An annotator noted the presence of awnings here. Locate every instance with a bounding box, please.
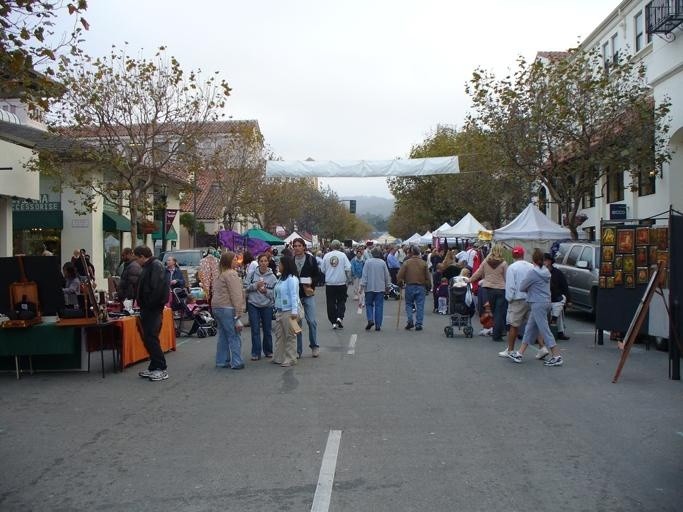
[137,220,178,241]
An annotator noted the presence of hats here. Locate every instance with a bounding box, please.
[512,246,526,257]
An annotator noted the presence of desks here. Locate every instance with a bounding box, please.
[0,302,176,382]
[591,285,668,353]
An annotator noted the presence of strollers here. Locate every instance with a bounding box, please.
[444,275,474,338]
[433,276,450,315]
[383,281,399,300]
[172,287,216,338]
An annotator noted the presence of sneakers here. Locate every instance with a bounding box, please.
[499,348,513,357]
[508,351,522,363]
[365,320,382,331]
[216,346,320,370]
[138,369,169,381]
[405,322,423,330]
[536,346,549,359]
[543,356,564,366]
[332,317,343,329]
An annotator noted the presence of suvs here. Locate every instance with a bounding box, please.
[161,246,217,289]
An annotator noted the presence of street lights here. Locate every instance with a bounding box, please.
[159,182,167,252]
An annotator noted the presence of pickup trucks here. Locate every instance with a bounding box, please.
[550,241,643,322]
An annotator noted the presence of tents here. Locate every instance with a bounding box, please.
[376,202,571,245]
[284,231,313,248]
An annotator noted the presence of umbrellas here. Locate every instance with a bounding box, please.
[238,226,285,247]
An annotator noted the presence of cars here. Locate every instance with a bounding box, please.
[271,243,293,265]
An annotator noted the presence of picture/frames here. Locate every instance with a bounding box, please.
[595,223,671,292]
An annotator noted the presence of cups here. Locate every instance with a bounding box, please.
[259,276,264,289]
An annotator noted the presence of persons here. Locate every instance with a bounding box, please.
[130,245,172,383]
[113,248,140,311]
[199,238,320,370]
[32,242,53,256]
[499,247,570,367]
[166,257,214,323]
[315,238,511,343]
[61,249,97,308]
